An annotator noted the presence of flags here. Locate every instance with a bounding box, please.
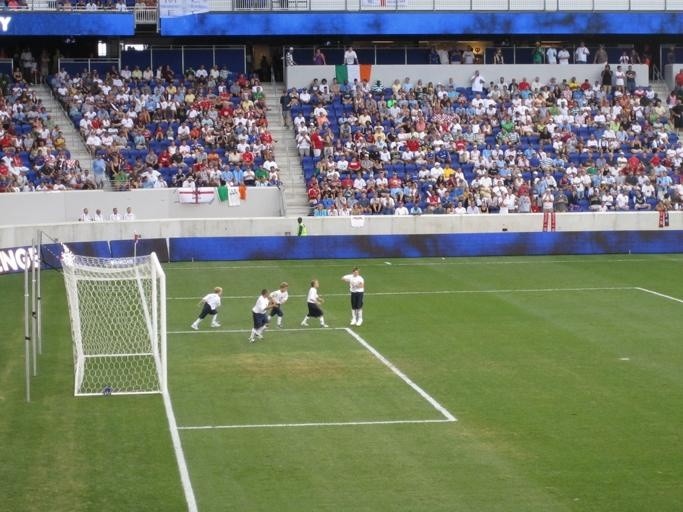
[217,185,247,201]
[177,186,216,204]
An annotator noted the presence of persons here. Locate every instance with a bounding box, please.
[267,282,288,329]
[123,207,136,220]
[189,286,222,329]
[280,65,682,216]
[1,1,159,21]
[78,207,93,222]
[248,288,278,343]
[296,217,308,236]
[300,278,330,330]
[1,46,284,193]
[109,207,122,221]
[341,268,365,326]
[92,209,105,221]
[286,40,678,65]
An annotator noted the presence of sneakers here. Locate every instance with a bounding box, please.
[350,319,363,326]
[248,333,265,343]
[321,324,328,328]
[211,322,220,327]
[277,324,282,327]
[301,321,309,327]
[191,323,199,329]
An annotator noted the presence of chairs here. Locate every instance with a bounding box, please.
[1,68,274,190]
[286,83,683,217]
